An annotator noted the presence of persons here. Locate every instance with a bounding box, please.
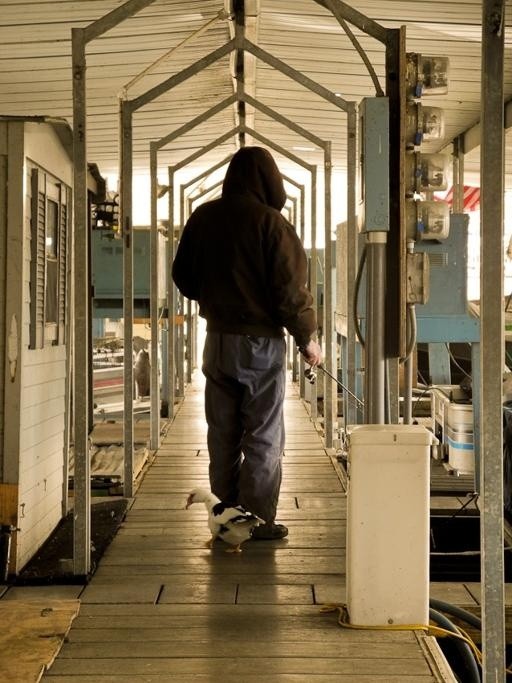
[171,146,325,540]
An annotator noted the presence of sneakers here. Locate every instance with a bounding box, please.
[253,523,288,540]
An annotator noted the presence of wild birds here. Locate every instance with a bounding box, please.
[184,486,264,553]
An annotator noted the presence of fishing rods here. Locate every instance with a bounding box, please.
[298,347,365,410]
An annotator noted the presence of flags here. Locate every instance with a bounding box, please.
[444,181,481,210]
